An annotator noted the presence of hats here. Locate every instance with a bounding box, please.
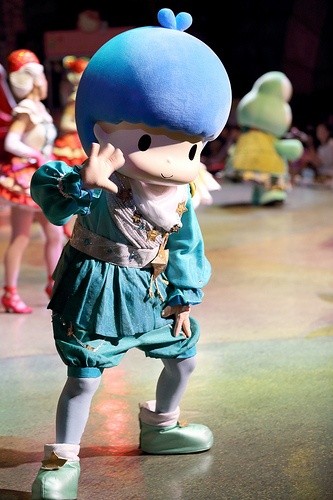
[8,49,39,71]
[64,55,90,74]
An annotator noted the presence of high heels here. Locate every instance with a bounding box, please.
[2,286,31,313]
[45,275,54,299]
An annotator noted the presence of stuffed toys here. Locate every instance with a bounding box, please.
[30,8,214,500]
[225,71,304,203]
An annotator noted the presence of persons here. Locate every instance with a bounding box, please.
[2,50,63,314]
[53,56,85,236]
[306,124,333,180]
[204,127,313,182]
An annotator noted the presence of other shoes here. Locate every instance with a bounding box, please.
[32,444,81,500]
[253,189,284,204]
[139,401,214,453]
